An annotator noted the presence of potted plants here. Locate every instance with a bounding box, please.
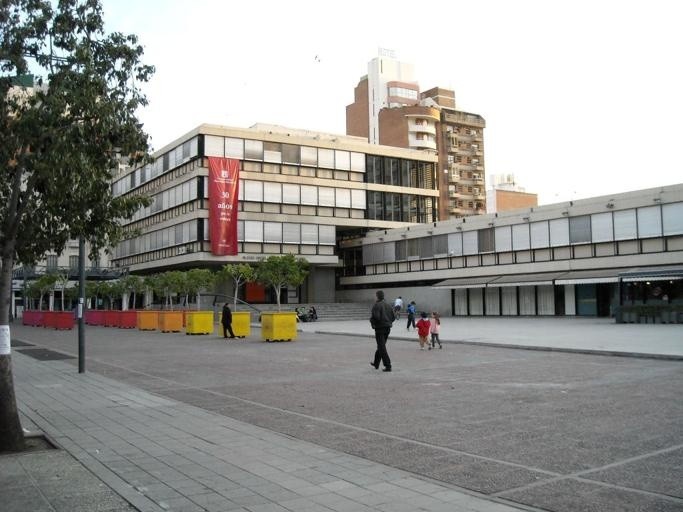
[19,251,310,342]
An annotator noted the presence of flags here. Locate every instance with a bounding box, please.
[207,155,242,259]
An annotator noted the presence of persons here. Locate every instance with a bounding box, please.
[406,301,417,331]
[415,311,432,350]
[370,290,396,373]
[296,306,308,322]
[429,312,443,349]
[221,302,237,338]
[394,296,403,320]
[308,306,317,322]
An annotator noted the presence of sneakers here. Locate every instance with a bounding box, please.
[419,344,442,350]
[383,368,390,372]
[371,363,378,369]
